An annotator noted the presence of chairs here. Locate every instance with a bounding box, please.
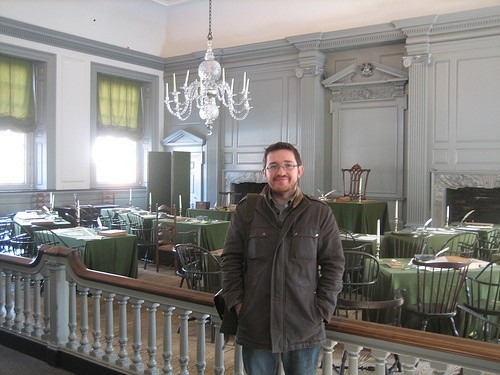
[0,164,500,375]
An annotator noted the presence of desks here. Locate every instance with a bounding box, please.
[56,205,119,227]
[185,208,233,221]
[320,198,390,235]
[442,222,500,250]
[33,228,138,279]
[99,208,178,243]
[149,217,231,266]
[363,254,500,343]
[12,212,72,235]
[339,233,388,266]
[387,221,479,258]
[202,249,224,294]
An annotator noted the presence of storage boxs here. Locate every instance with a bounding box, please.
[196,202,210,210]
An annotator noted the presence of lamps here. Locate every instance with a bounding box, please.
[164,0,251,134]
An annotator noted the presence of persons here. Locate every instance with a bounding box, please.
[222,142,345,375]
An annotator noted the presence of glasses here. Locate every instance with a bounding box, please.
[265,162,299,172]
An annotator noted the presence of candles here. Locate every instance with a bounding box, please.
[50,192,52,203]
[179,194,182,208]
[129,188,132,200]
[377,219,380,244]
[77,200,80,217]
[149,192,152,204]
[52,195,55,208]
[394,200,398,218]
[359,178,362,194]
[446,206,449,217]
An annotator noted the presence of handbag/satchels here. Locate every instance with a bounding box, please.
[214,288,225,320]
